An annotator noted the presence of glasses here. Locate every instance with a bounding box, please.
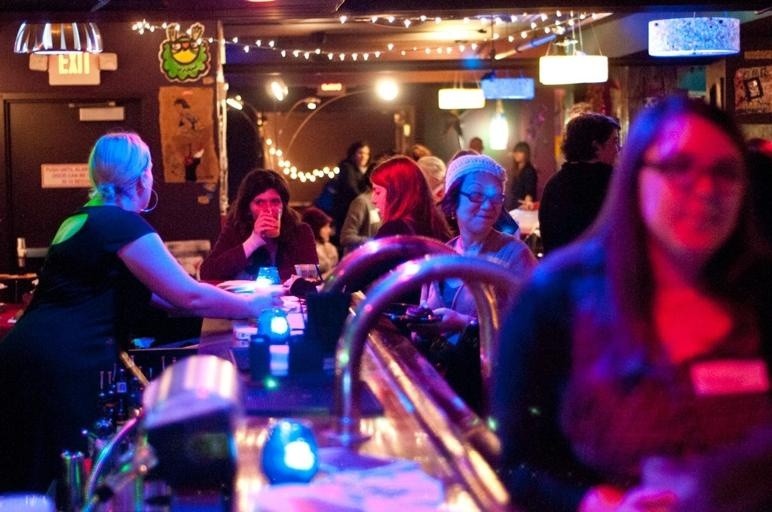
[641,158,746,192]
[459,190,507,205]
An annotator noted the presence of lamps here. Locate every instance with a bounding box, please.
[647,12,740,57]
[437,34,485,110]
[375,77,398,101]
[13,20,103,55]
[481,17,535,100]
[265,76,287,101]
[538,13,608,86]
[316,80,346,97]
[304,98,320,110]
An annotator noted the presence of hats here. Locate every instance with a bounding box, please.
[301,207,333,243]
[439,151,508,199]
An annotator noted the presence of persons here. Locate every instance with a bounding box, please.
[173,97,207,183]
[400,150,542,420]
[537,112,622,257]
[199,168,319,286]
[509,142,537,211]
[340,154,396,258]
[286,157,455,338]
[470,137,485,154]
[408,145,430,164]
[416,155,460,237]
[330,141,373,262]
[1,129,288,492]
[300,207,340,281]
[487,95,770,511]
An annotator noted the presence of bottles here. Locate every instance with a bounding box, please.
[257,307,290,344]
[258,266,282,284]
[259,413,321,485]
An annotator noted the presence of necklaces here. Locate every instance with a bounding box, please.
[459,236,482,253]
